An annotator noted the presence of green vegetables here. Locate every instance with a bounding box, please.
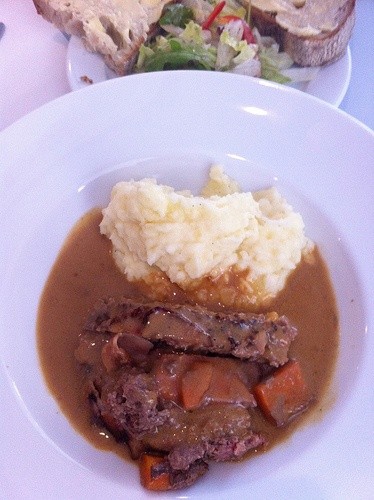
[133,0,291,84]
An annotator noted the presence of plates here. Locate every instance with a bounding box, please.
[0,70,374,498]
[65,33,353,109]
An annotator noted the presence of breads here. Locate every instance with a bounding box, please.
[31,0,175,75]
[238,0,355,66]
